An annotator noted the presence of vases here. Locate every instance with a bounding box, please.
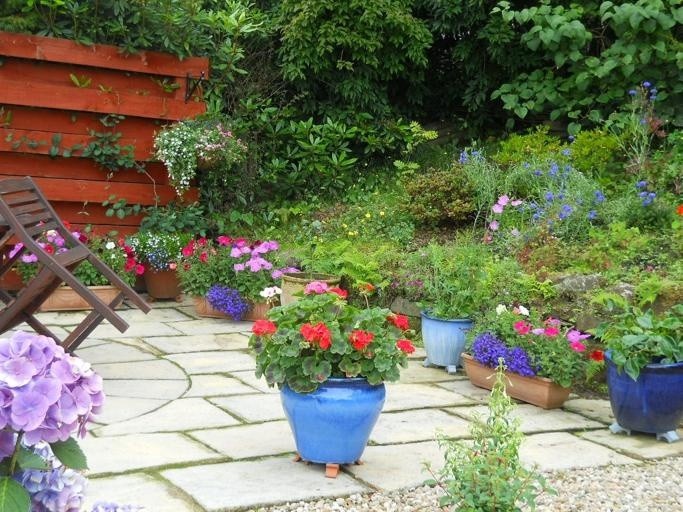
[460,352,571,408]
[197,156,222,169]
[279,377,385,464]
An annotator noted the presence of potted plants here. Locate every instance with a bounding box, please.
[585,293,683,443]
[408,230,498,374]
[281,223,390,314]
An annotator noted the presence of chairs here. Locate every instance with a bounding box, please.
[0,176,151,358]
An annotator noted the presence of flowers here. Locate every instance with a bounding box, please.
[472,302,605,385]
[154,113,250,198]
[248,281,415,394]
[8,221,300,320]
